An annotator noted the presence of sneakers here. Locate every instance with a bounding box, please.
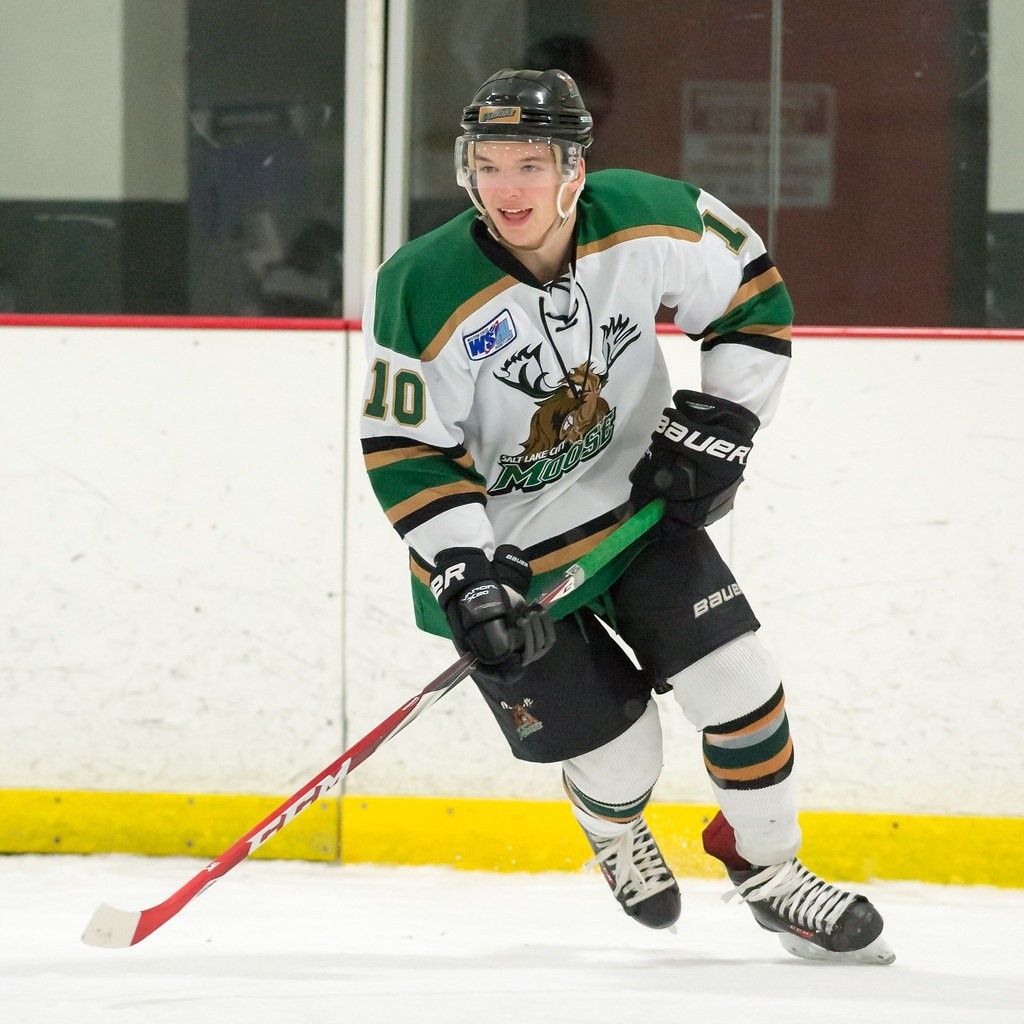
[703,807,895,967]
[567,790,682,935]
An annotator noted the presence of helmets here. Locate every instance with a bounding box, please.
[460,67,594,152]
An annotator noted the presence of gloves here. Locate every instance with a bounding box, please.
[630,388,761,544]
[428,542,553,687]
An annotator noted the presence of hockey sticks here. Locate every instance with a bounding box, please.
[75,491,670,962]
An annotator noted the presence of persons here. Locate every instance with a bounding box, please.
[359,68,885,956]
